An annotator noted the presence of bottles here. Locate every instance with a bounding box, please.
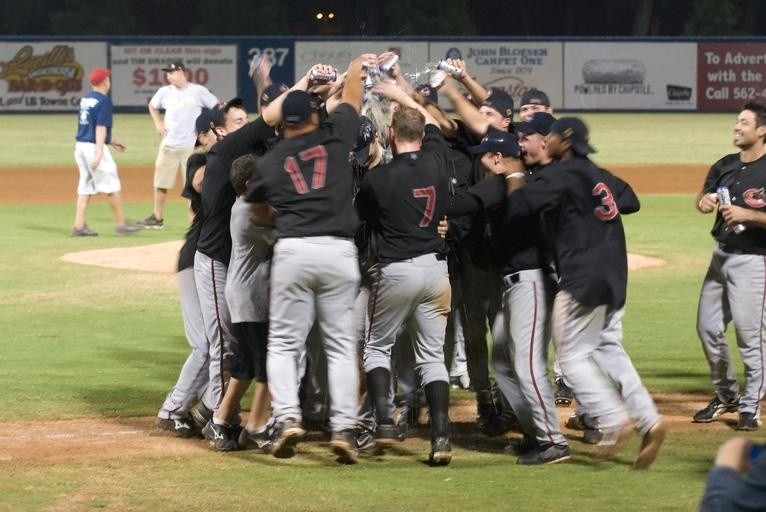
[373,64,380,77]
[311,68,336,81]
[381,55,399,71]
[364,75,373,86]
[732,222,745,234]
[437,59,464,79]
[717,186,732,207]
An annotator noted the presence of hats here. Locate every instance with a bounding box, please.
[281,90,325,126]
[520,90,550,107]
[351,115,375,164]
[89,68,112,86]
[260,83,289,107]
[416,83,439,104]
[208,97,243,135]
[196,112,220,134]
[479,87,514,119]
[162,62,185,71]
[509,112,556,135]
[469,131,521,157]
[551,117,596,156]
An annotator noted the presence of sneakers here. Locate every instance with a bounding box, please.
[737,412,762,430]
[633,420,667,469]
[556,385,572,408]
[155,409,196,438]
[396,402,418,427]
[239,427,276,453]
[331,429,355,466]
[194,407,214,427]
[271,418,306,459]
[72,224,97,237]
[201,420,237,451]
[357,433,375,451]
[137,214,165,232]
[478,405,495,430]
[692,398,742,424]
[485,410,517,435]
[505,436,540,455]
[429,435,453,465]
[113,223,144,233]
[517,443,571,466]
[593,423,636,462]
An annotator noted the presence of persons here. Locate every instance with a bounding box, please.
[358,82,457,467]
[252,53,378,466]
[136,60,217,230]
[513,110,605,444]
[199,153,296,455]
[428,58,523,434]
[445,129,573,468]
[193,63,338,442]
[151,108,221,440]
[505,116,669,472]
[692,101,766,432]
[518,89,556,130]
[343,107,395,439]
[71,67,144,236]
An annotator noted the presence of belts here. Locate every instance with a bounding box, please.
[409,252,448,263]
[720,243,764,258]
[501,274,524,288]
